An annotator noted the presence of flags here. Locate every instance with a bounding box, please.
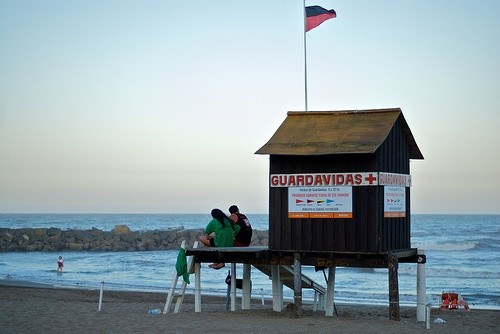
[306,5,336,32]
[175,248,191,284]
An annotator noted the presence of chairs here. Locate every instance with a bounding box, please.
[438,290,465,310]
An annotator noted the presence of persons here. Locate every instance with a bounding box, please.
[200,209,240,247]
[209,206,252,270]
[57,256,64,271]
